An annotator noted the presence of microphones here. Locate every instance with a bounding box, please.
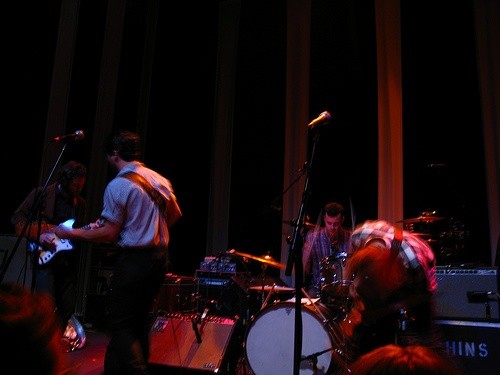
[51,131,85,143]
[192,324,203,343]
[308,111,332,129]
[466,290,496,301]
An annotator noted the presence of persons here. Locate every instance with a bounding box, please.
[336,219,438,335]
[301,204,357,298]
[13,162,93,327]
[56,132,176,375]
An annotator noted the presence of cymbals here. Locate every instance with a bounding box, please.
[397,217,443,224]
[228,250,285,269]
[249,285,294,291]
[411,232,429,236]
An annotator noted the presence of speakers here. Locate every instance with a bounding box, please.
[436,266,499,322]
[148,311,248,375]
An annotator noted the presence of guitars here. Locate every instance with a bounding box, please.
[29,218,104,265]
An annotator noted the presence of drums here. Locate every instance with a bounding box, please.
[245,300,344,375]
[319,253,358,297]
